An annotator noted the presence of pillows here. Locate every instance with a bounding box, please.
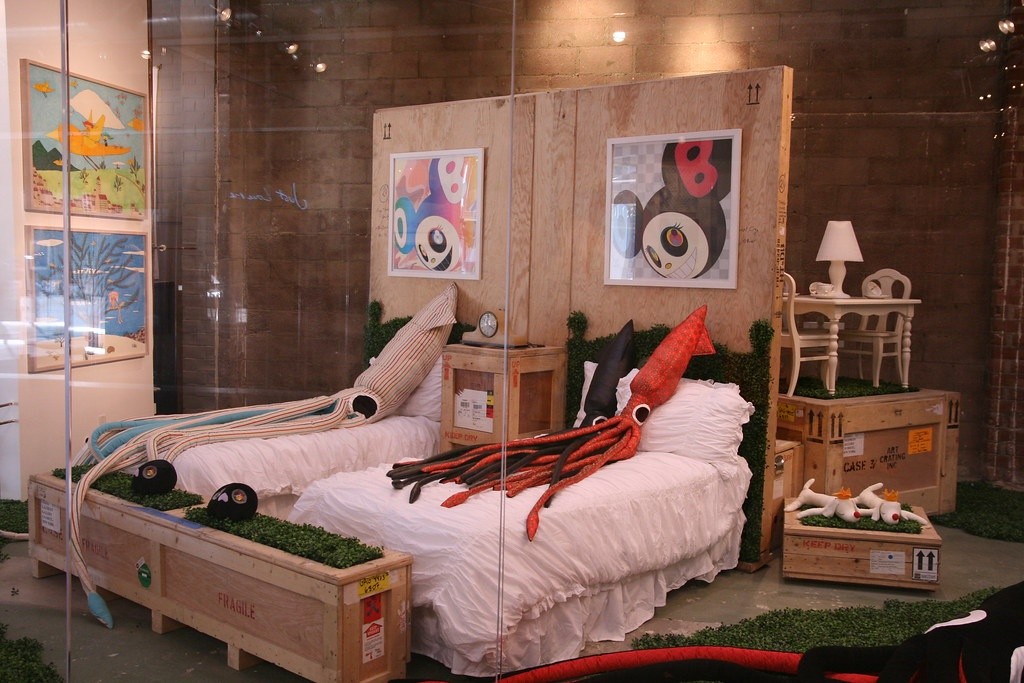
[617,370,753,484]
[573,358,629,427]
[386,352,444,420]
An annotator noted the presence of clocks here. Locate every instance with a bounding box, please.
[461,307,529,346]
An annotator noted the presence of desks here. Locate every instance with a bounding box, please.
[784,293,923,394]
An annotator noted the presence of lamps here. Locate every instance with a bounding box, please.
[208,3,327,74]
[815,219,863,300]
[979,5,1024,53]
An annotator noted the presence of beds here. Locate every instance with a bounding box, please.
[71,299,478,520]
[288,311,775,676]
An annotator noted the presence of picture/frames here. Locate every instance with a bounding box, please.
[23,224,151,375]
[386,147,485,280]
[19,58,150,221]
[602,128,743,290]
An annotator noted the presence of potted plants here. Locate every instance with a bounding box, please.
[27,470,413,682]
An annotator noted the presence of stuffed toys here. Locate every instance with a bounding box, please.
[385,304,716,542]
[132,459,178,499]
[386,581,1024,683]
[69,282,460,631]
[207,482,259,524]
[783,478,928,525]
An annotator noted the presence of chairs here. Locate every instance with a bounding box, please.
[782,269,837,397]
[836,269,912,391]
